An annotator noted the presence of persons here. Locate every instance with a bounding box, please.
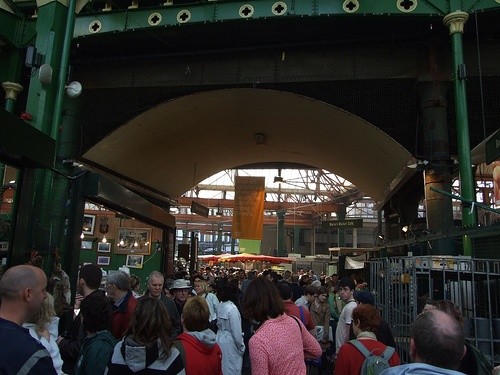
[21,259,500,375]
[377,309,467,375]
[0,264,58,375]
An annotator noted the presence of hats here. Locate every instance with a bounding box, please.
[325,276,331,279]
[359,290,374,304]
[280,284,290,291]
[169,279,192,295]
[320,276,324,278]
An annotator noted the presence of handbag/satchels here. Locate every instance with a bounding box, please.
[307,355,321,375]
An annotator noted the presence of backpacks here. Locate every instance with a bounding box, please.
[347,340,396,375]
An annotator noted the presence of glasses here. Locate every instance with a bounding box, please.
[106,282,117,286]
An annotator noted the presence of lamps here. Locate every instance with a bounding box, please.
[274,169,283,183]
[255,133,264,145]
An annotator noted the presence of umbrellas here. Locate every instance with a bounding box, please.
[197,252,293,263]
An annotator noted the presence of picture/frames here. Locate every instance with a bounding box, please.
[126,254,144,269]
[113,227,152,255]
[97,256,110,266]
[97,242,111,252]
[80,240,93,249]
[81,214,96,236]
